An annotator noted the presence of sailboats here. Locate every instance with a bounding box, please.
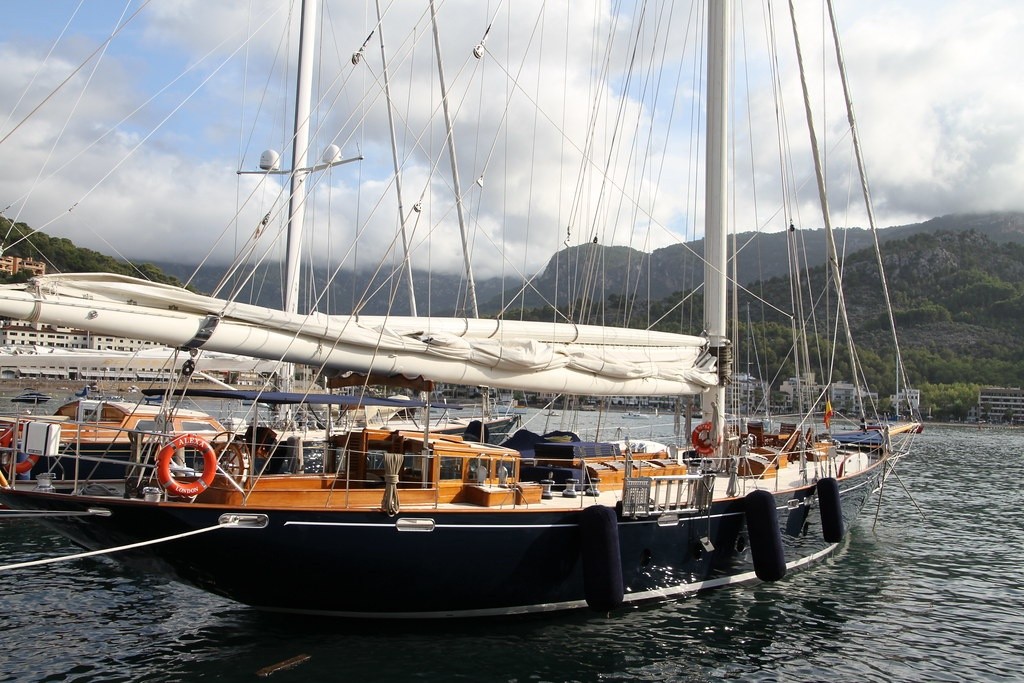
[0,0,921,624]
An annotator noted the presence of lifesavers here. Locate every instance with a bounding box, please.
[692,423,722,455]
[0,422,40,473]
[155,432,218,498]
